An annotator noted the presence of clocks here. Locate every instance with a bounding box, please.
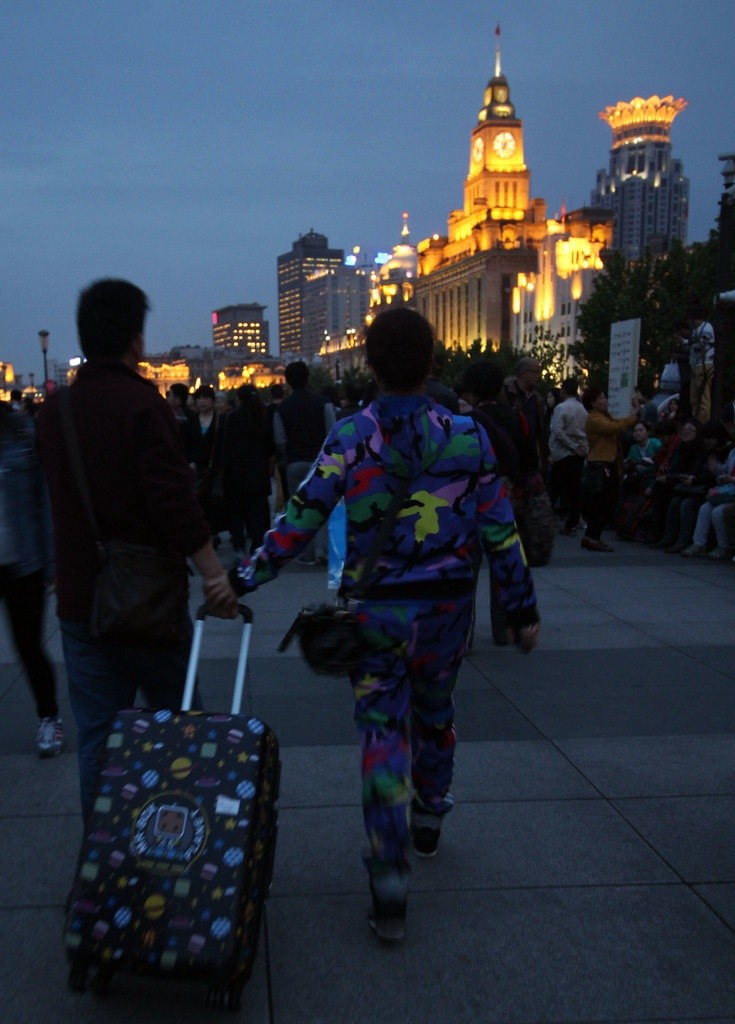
[493,131,517,158]
[471,137,484,163]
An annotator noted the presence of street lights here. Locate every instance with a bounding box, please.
[29,372,36,400]
[38,330,50,383]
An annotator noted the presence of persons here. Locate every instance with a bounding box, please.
[0,307,735,758]
[202,305,542,942]
[34,278,238,835]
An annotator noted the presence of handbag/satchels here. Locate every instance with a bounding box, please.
[279,607,372,680]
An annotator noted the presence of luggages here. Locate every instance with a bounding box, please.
[61,599,283,1015]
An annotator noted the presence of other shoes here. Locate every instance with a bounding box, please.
[617,521,735,565]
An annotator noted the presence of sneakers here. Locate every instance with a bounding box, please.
[582,535,612,549]
[297,548,327,567]
[406,818,442,856]
[366,905,405,943]
[30,716,69,757]
